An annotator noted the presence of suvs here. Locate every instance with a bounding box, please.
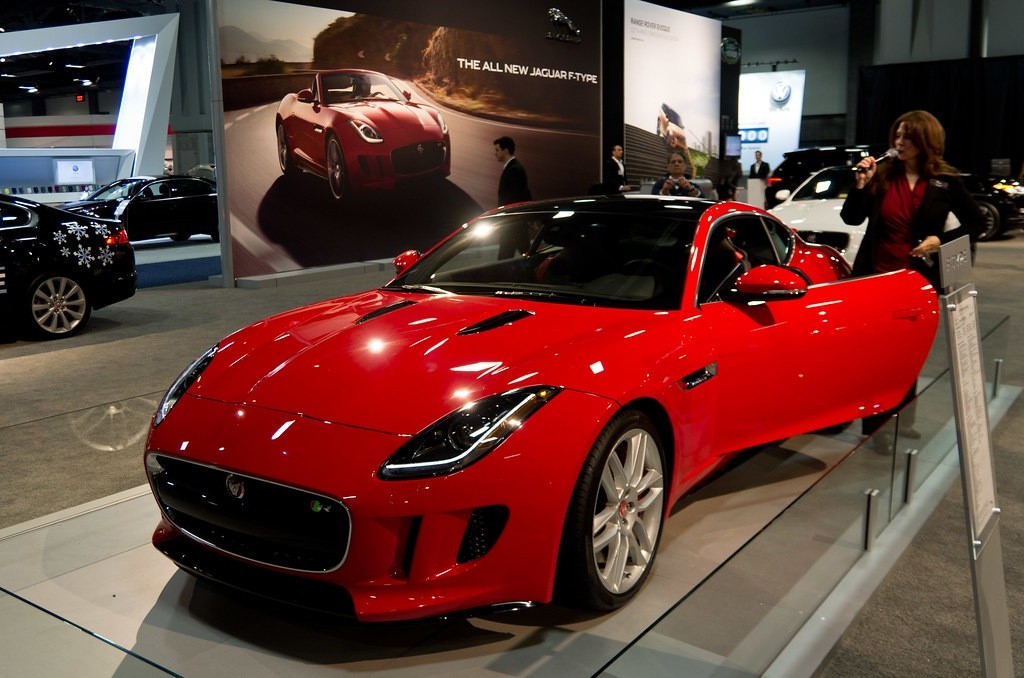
[656,102,688,152]
[764,144,1024,243]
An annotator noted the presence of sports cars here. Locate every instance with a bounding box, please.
[274,68,452,201]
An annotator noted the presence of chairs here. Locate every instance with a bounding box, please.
[143,186,155,199]
[159,185,167,197]
[654,219,752,301]
[532,232,610,285]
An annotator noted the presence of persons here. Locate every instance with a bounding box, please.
[840,111,988,455]
[716,163,742,201]
[493,137,532,260]
[651,153,705,198]
[749,151,770,179]
[605,144,631,194]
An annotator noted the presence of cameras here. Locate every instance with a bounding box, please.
[672,179,680,185]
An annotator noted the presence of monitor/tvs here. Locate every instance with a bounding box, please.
[724,133,741,159]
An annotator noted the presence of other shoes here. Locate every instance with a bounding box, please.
[898,424,921,440]
[873,428,893,456]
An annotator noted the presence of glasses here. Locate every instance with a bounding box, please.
[669,160,684,164]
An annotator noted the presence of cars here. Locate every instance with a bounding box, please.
[764,165,962,274]
[141,195,942,627]
[0,191,139,342]
[53,175,220,243]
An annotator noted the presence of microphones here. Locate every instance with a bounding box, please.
[857,148,899,173]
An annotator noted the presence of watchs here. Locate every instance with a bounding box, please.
[688,185,695,192]
